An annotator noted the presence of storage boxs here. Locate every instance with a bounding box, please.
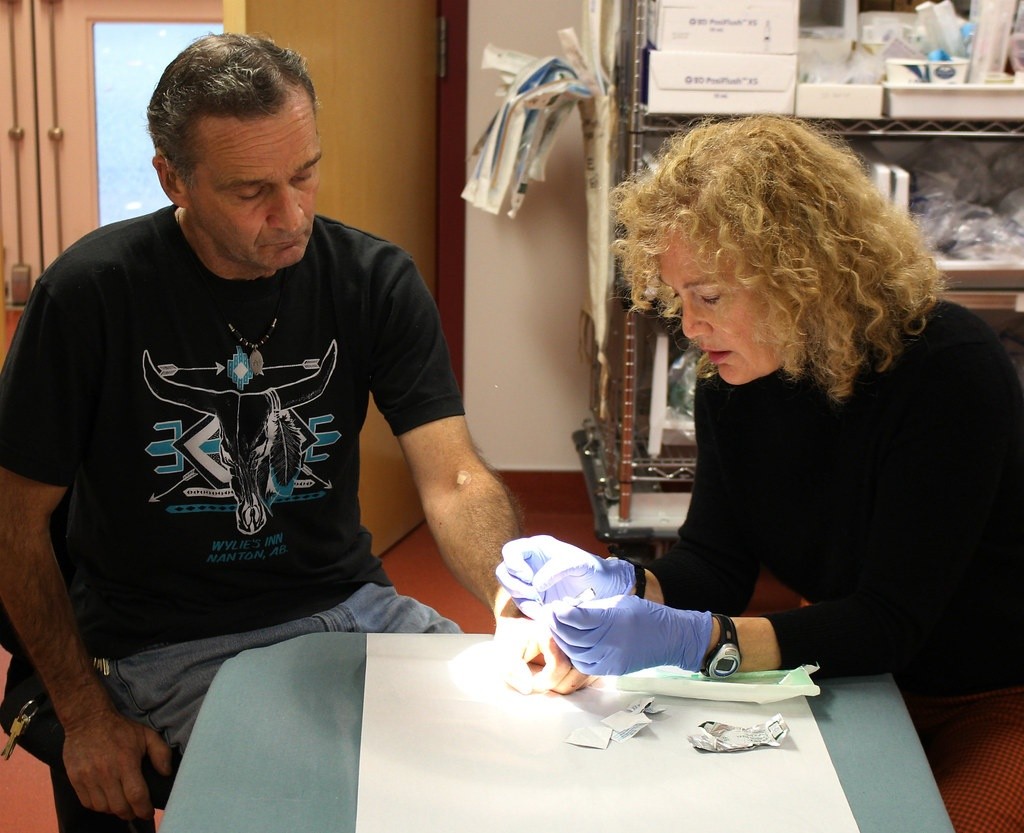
[643,0,1024,120]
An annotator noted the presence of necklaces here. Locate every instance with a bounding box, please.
[177,216,290,377]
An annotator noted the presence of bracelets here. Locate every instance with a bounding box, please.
[623,557,646,598]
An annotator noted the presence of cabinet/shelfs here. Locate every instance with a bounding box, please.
[596,0,1024,520]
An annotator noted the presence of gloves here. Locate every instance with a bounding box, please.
[551,595,713,675]
[496,534,636,624]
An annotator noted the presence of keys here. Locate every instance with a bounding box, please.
[1,715,31,760]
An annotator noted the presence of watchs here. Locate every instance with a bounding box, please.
[702,614,742,680]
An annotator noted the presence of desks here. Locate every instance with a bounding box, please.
[155,632,957,832]
[572,430,694,601]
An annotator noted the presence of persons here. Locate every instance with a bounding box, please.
[496,115,1024,833]
[0,32,600,820]
